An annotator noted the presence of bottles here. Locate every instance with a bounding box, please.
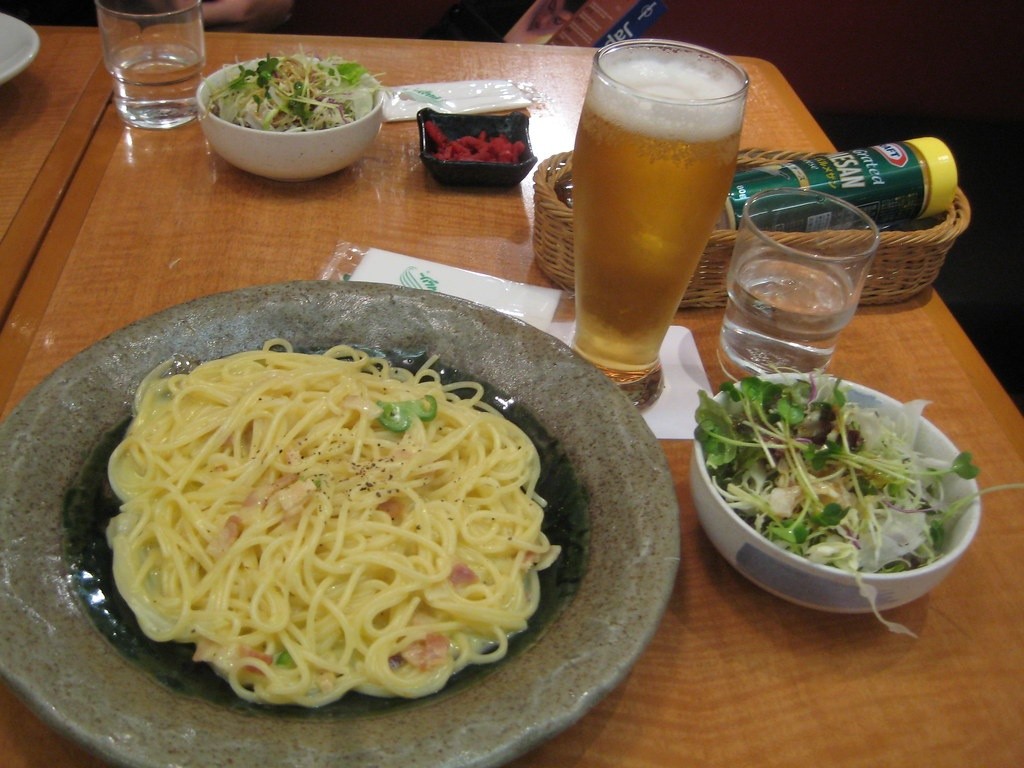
[725,136,957,226]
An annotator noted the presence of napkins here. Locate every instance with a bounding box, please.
[322,240,561,332]
[382,80,532,121]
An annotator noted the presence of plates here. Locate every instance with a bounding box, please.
[0,13,41,87]
[416,108,538,186]
[0,279,682,768]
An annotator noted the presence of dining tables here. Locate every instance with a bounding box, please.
[0,27,1024,768]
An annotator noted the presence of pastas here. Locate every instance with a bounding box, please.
[104,339,551,709]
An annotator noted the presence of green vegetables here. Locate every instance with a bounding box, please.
[198,41,387,133]
[693,373,1024,639]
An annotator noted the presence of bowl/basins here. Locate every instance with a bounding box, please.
[691,372,981,613]
[196,58,385,181]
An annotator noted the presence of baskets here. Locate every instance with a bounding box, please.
[533,147,971,307]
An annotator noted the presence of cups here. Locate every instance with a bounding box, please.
[95,0,205,130]
[716,187,881,382]
[572,39,751,407]
